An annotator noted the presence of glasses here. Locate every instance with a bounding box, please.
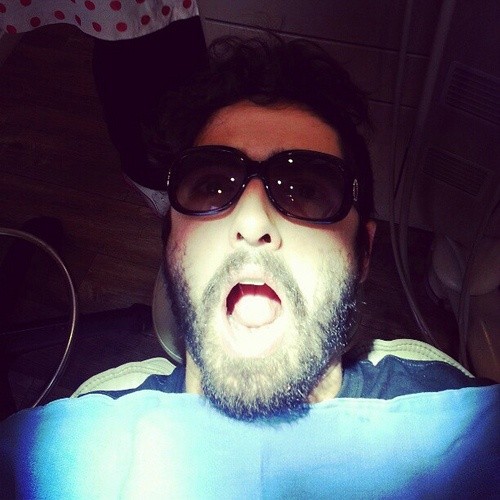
[155,145,365,224]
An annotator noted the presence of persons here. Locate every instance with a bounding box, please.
[3,28,500,500]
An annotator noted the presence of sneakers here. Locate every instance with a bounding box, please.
[120,171,172,215]
